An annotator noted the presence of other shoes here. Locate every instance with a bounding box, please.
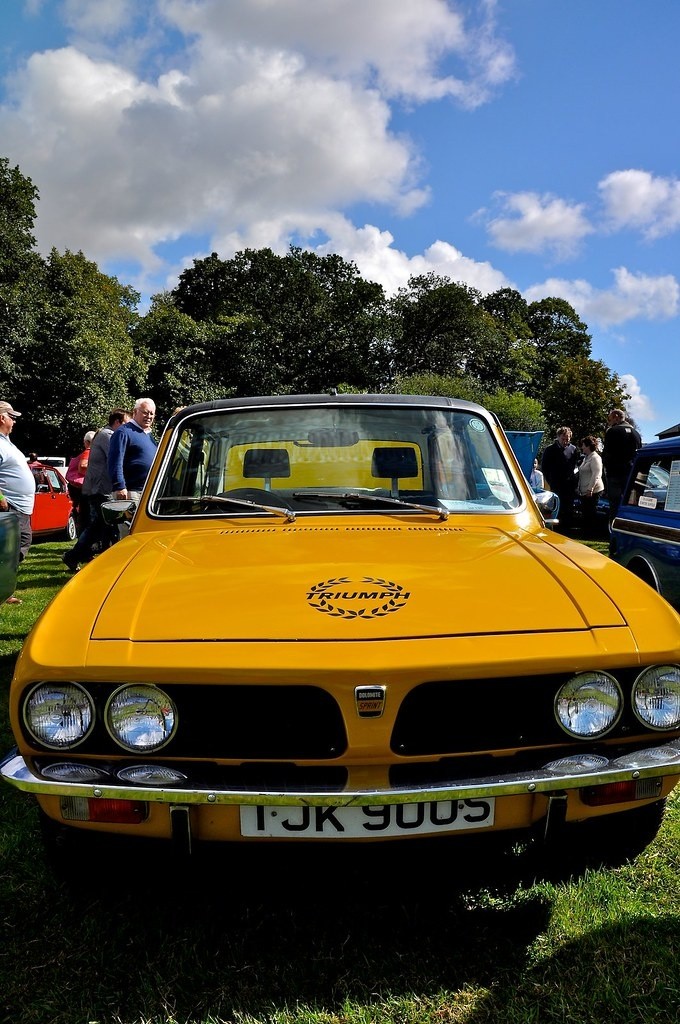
[62,552,81,573]
[7,597,22,604]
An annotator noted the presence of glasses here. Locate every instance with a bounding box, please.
[5,413,17,421]
[137,408,157,417]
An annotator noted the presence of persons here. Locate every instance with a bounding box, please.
[62,398,210,572]
[530,458,544,490]
[579,437,605,541]
[603,409,642,523]
[541,427,579,538]
[0,401,43,605]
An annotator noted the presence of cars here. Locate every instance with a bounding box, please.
[573,496,610,533]
[608,436,680,615]
[23,464,77,544]
[22,456,67,472]
[0,391,680,894]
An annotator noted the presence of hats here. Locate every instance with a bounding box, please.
[0,401,21,417]
[29,453,37,460]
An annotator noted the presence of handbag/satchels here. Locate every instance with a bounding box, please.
[66,458,87,486]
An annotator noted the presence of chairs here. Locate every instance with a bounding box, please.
[533,489,560,530]
[371,447,419,498]
[243,449,290,490]
